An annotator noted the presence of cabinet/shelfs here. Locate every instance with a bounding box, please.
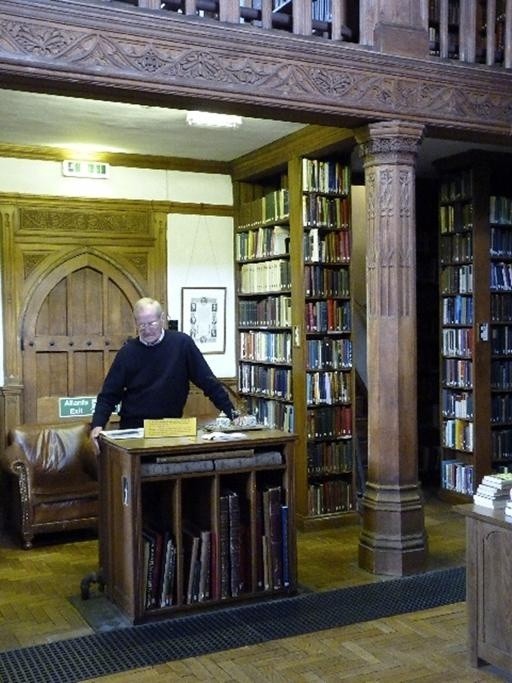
[229,157,362,535]
[451,498,512,683]
[433,149,511,506]
[97,418,300,625]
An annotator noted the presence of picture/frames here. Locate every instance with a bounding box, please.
[179,283,228,357]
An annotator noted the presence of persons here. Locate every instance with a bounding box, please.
[86,295,237,457]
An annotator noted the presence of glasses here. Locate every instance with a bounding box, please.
[135,321,159,328]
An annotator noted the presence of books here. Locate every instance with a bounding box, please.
[434,170,510,498]
[235,188,296,434]
[301,160,360,515]
[471,472,512,520]
[428,1,509,65]
[131,446,290,608]
[271,1,334,31]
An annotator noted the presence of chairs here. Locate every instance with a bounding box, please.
[2,422,100,552]
